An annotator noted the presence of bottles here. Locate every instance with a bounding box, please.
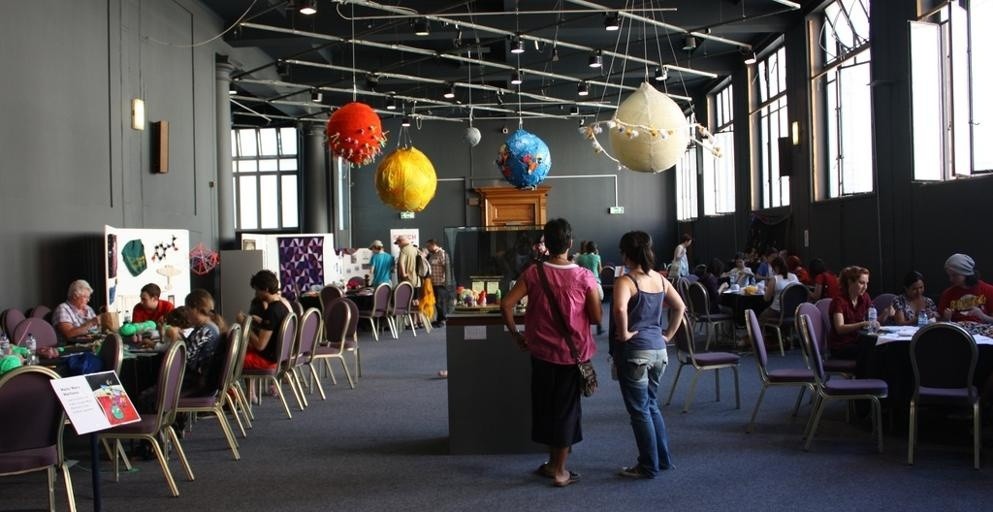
[868,304,878,335]
[0,334,9,358]
[27,333,36,366]
[730,272,736,289]
[918,310,927,330]
[751,275,756,285]
[124,310,131,323]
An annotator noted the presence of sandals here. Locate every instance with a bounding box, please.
[406,324,419,330]
[417,321,425,329]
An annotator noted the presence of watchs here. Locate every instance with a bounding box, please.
[512,330,520,338]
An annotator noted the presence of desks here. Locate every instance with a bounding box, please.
[445,309,572,455]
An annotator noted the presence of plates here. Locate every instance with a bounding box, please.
[897,331,913,336]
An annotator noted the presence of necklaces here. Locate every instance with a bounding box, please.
[550,255,568,261]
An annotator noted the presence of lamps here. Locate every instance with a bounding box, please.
[411,18,431,36]
[299,1,318,15]
[508,38,525,54]
[589,55,602,68]
[605,13,620,32]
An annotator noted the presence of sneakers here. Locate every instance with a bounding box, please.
[620,464,658,478]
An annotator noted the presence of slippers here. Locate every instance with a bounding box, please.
[537,464,583,488]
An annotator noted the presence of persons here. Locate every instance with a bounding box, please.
[134,288,228,457]
[755,257,800,344]
[827,265,895,359]
[394,235,424,330]
[673,234,693,277]
[578,240,606,334]
[807,259,842,298]
[894,271,939,323]
[53,278,102,339]
[418,246,433,262]
[425,237,449,327]
[501,216,604,486]
[785,255,813,286]
[236,270,290,371]
[745,246,774,278]
[609,230,686,479]
[726,252,755,287]
[937,252,993,323]
[131,283,175,320]
[369,240,395,289]
[698,258,734,345]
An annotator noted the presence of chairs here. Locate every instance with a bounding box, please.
[752,262,761,273]
[358,283,395,341]
[193,316,254,428]
[664,263,666,270]
[292,301,355,394]
[688,282,736,351]
[181,323,246,448]
[695,264,708,277]
[97,340,195,497]
[382,281,416,339]
[799,314,887,453]
[909,324,979,469]
[317,298,362,384]
[30,305,53,323]
[2,308,26,343]
[264,300,308,393]
[666,307,741,413]
[176,329,240,461]
[666,263,672,271]
[64,333,133,471]
[873,294,897,317]
[13,317,58,348]
[745,309,816,435]
[759,281,809,357]
[259,307,326,407]
[791,303,856,423]
[0,365,78,512]
[677,277,691,306]
[815,298,832,360]
[600,266,615,287]
[235,312,304,419]
[399,278,432,334]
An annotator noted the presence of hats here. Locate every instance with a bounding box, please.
[395,234,408,244]
[368,239,384,249]
